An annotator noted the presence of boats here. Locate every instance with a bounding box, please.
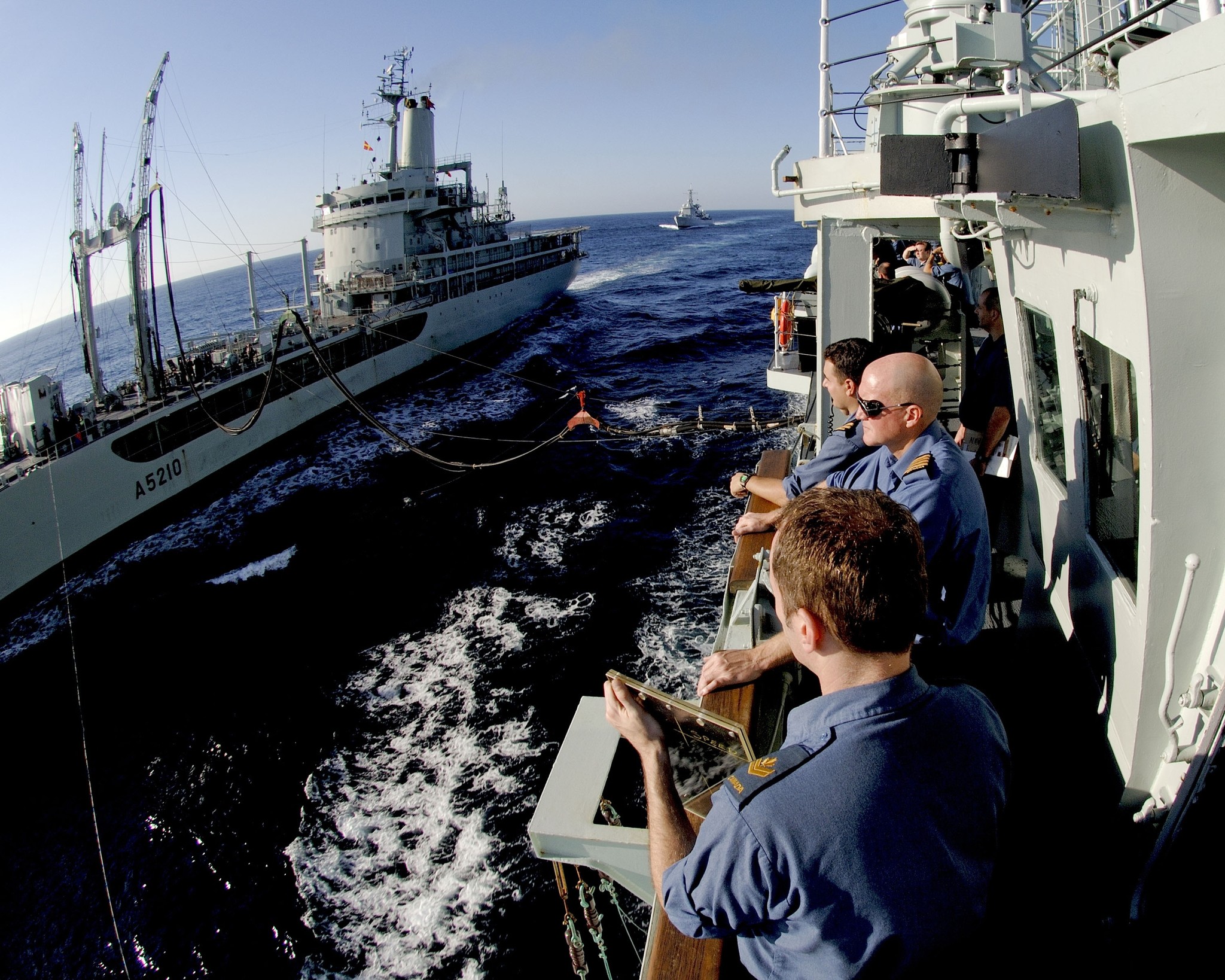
[673,183,715,227]
[2,43,587,600]
[528,0,1224,980]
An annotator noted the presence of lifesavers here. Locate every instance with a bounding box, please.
[779,298,794,350]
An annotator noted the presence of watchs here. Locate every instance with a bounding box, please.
[740,473,756,489]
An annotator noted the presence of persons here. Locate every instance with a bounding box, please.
[438,187,455,204]
[730,338,884,508]
[954,287,1018,602]
[43,343,256,446]
[696,352,991,697]
[603,488,1010,980]
[873,237,970,334]
[363,179,373,205]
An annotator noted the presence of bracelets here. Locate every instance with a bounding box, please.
[975,452,988,462]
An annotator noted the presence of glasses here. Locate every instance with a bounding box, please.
[855,386,924,419]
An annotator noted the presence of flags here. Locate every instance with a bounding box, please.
[427,98,436,109]
[364,140,374,151]
[405,97,414,108]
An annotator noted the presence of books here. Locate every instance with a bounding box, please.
[960,428,1019,478]
